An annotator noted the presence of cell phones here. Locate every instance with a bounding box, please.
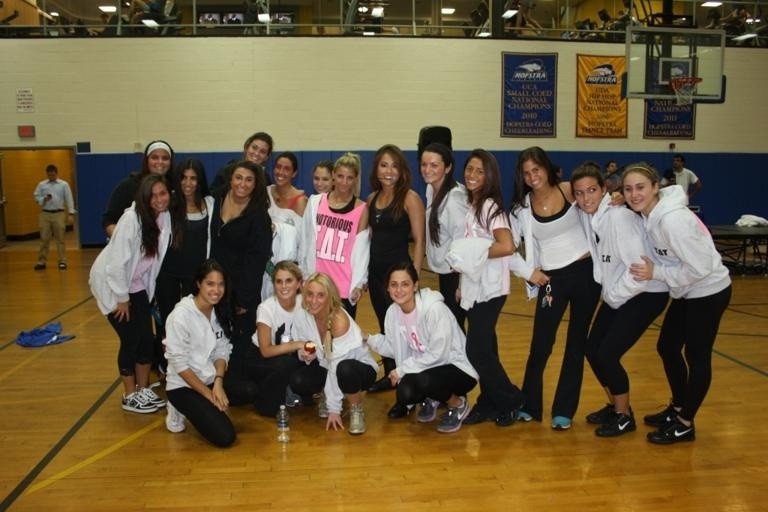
[48,195,51,198]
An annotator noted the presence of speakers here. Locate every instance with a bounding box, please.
[418,126,451,174]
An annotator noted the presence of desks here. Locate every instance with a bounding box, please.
[708,224,768,278]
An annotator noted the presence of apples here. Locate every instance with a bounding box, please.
[304,341,317,354]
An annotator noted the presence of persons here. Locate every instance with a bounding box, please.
[33,165,77,269]
[705,4,752,35]
[455,146,730,444]
[93,13,110,37]
[382,27,400,34]
[127,0,151,31]
[504,0,544,34]
[90,130,480,446]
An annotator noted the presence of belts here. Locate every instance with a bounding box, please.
[42,209,64,213]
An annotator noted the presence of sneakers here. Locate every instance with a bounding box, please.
[436,395,470,433]
[417,397,440,422]
[586,403,695,445]
[122,381,165,414]
[318,398,329,418]
[462,403,532,426]
[367,376,392,392]
[58,263,67,269]
[166,400,186,432]
[35,263,46,270]
[551,416,572,430]
[387,401,416,418]
[286,384,302,408]
[349,402,367,434]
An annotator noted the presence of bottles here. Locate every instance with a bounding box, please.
[275,404,291,443]
